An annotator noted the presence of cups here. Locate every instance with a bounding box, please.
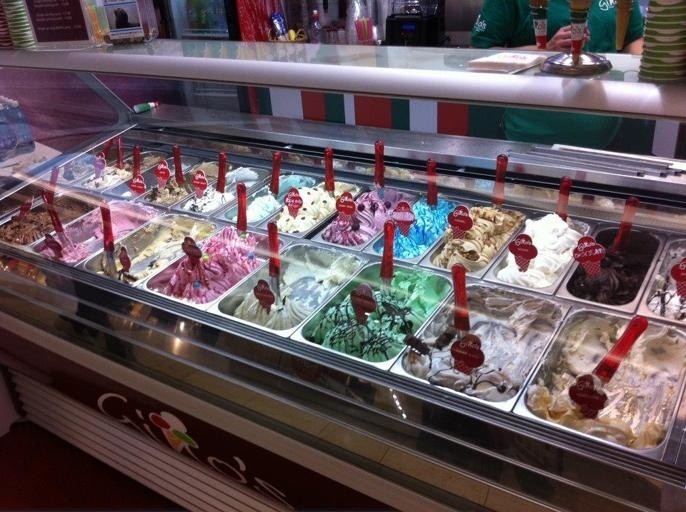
[637,0,686,84]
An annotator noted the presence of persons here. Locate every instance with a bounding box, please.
[469,0,644,148]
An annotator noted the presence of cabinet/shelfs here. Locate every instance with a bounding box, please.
[1,40,685,511]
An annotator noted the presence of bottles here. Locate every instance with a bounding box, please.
[0,94,35,162]
[133,101,159,113]
[311,10,322,44]
